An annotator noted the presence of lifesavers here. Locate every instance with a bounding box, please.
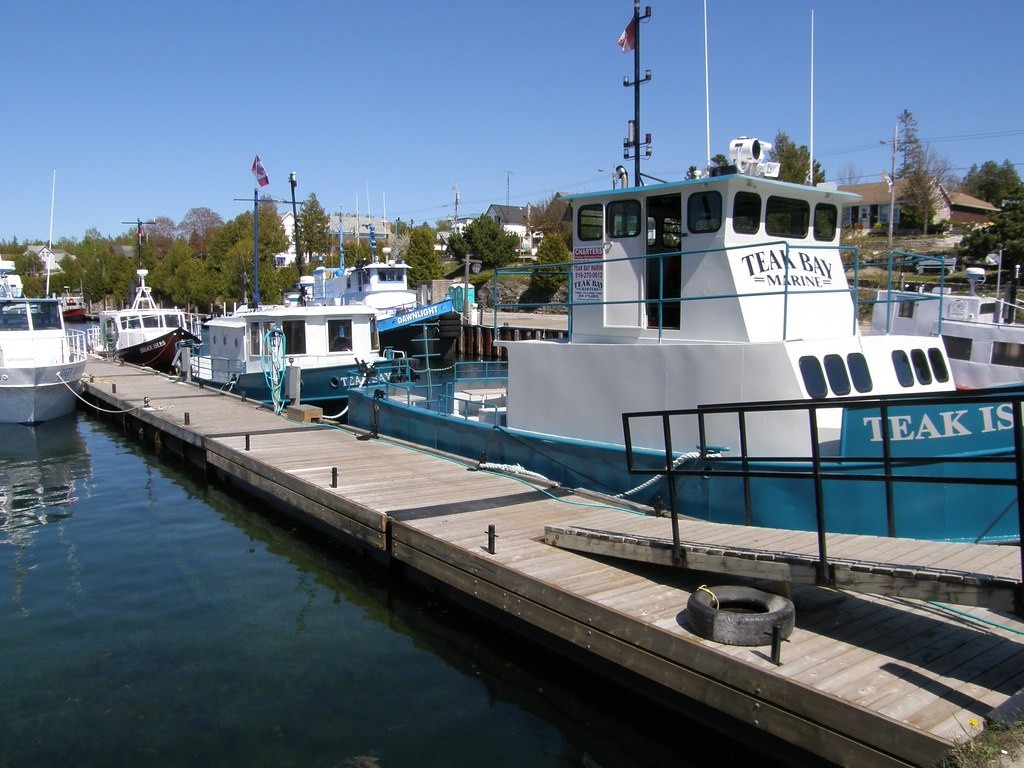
[687,585,796,646]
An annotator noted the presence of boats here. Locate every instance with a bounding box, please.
[0,249,88,426]
[172,0,1023,559]
[56,285,86,322]
[86,268,204,374]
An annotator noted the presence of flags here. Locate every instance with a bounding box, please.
[252,155,269,188]
[617,16,637,55]
